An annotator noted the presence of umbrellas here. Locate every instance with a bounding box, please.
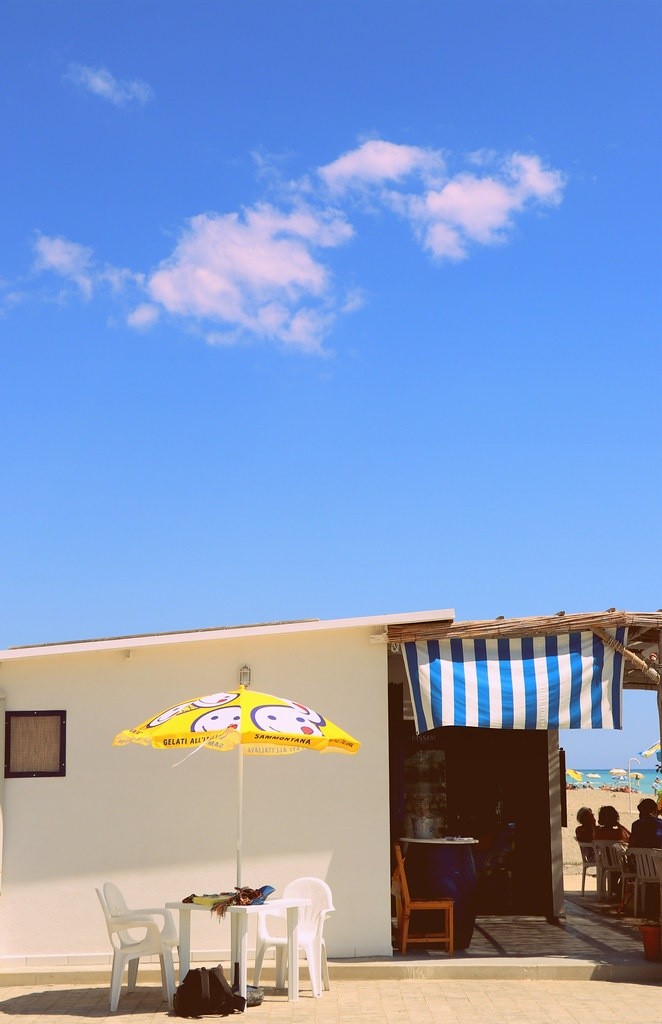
[566,769,582,784]
[609,768,627,777]
[628,772,644,779]
[611,776,628,781]
[586,773,601,786]
[112,684,362,1012]
[637,739,662,762]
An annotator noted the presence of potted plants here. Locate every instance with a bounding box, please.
[639,913,662,962]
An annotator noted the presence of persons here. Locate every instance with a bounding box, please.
[575,806,600,862]
[602,784,640,793]
[632,798,662,849]
[587,780,594,789]
[593,806,631,895]
[655,776,660,796]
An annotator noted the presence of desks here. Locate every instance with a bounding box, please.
[399,838,479,950]
[165,897,308,1010]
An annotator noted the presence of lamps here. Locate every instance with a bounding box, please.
[239,665,252,687]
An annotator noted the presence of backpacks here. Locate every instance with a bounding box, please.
[172,964,234,1017]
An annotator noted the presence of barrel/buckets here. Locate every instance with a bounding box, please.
[411,816,434,839]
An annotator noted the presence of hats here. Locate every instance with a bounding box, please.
[252,886,275,905]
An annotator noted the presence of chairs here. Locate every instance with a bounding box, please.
[253,877,336,998]
[573,836,662,917]
[95,882,198,1014]
[393,842,456,957]
